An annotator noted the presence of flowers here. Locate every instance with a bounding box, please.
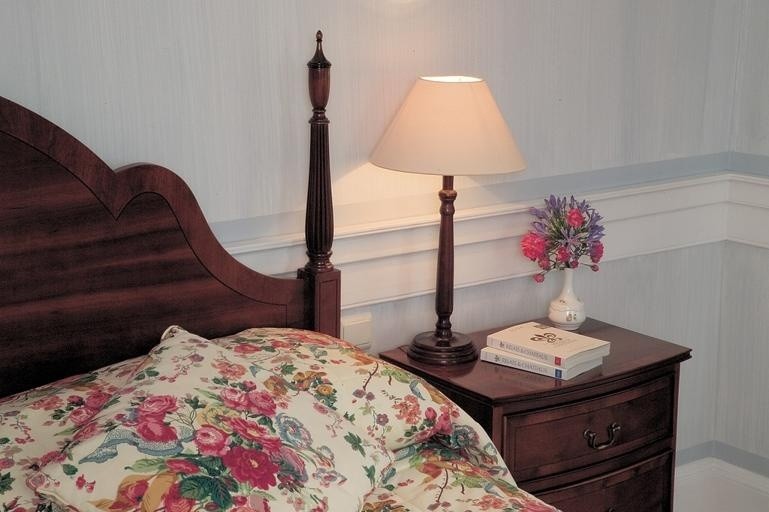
[521,194,606,283]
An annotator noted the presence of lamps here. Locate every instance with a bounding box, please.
[368,76,527,366]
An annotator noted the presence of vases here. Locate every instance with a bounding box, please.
[548,268,586,330]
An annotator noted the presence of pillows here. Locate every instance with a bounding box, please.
[0,328,514,512]
[26,325,395,512]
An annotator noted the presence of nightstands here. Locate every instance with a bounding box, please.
[379,317,693,512]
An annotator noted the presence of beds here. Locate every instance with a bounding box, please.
[0,31,561,512]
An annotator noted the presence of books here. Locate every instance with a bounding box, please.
[480,345,603,382]
[485,322,612,370]
